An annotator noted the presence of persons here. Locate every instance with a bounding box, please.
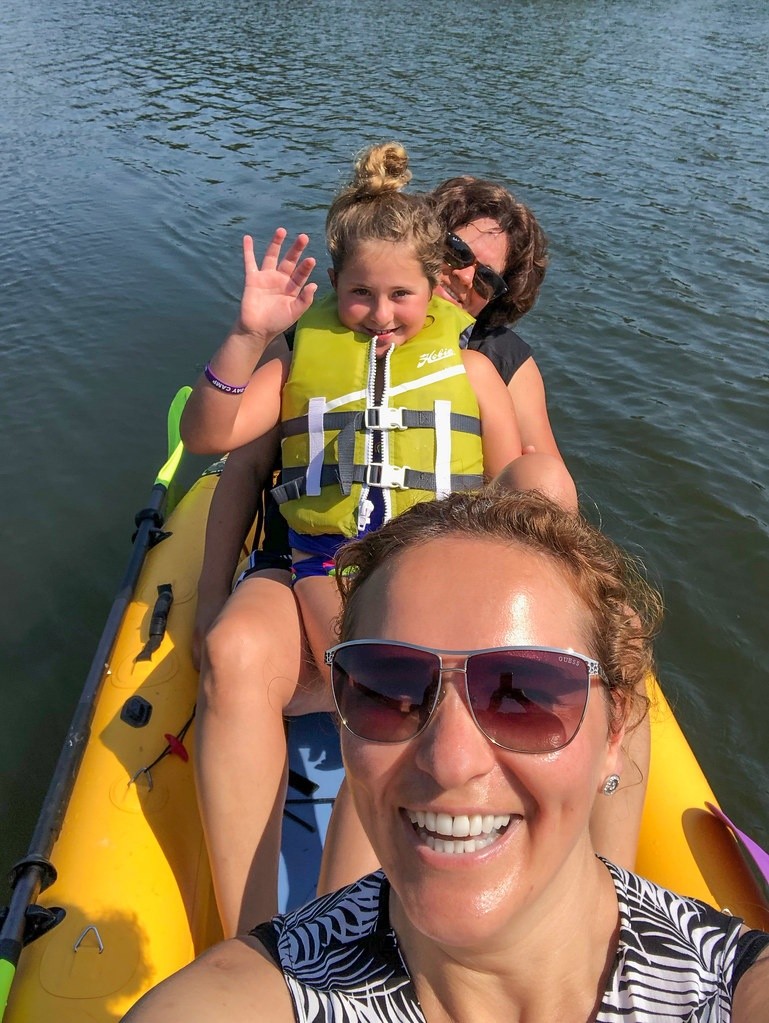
[188,172,582,701]
[178,141,526,944]
[117,489,769,1023]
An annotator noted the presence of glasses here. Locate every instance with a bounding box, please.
[324,637,611,754]
[442,232,511,300]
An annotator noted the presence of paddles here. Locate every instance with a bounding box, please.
[705,801,769,906]
[0,385,192,1022]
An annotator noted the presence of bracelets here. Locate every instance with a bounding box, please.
[204,358,248,397]
